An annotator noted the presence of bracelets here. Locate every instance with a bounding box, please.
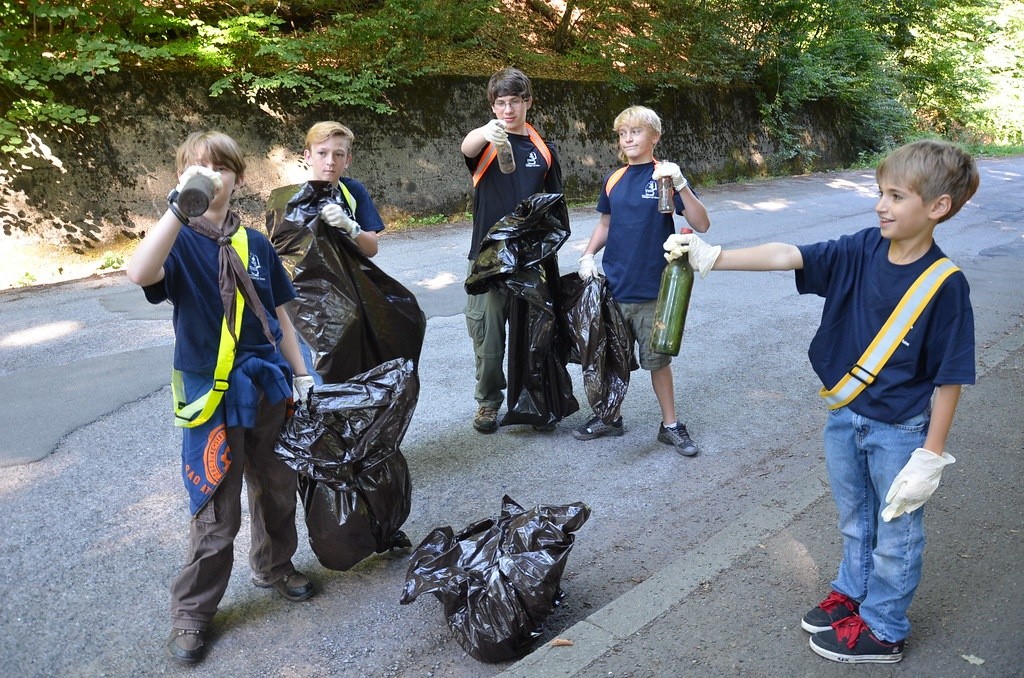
[168,202,190,227]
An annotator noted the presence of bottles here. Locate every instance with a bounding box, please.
[648,227,695,356]
[494,140,516,174]
[657,159,677,215]
[177,174,215,216]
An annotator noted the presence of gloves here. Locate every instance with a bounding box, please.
[881,447,956,523]
[176,164,223,191]
[483,119,509,147]
[578,253,601,282]
[292,375,315,403]
[320,204,356,237]
[652,160,688,192]
[663,233,722,279]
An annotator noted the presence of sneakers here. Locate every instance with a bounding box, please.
[251,566,315,601]
[533,424,555,431]
[658,417,698,456]
[166,627,206,663]
[801,589,905,663]
[571,415,624,440]
[473,406,498,431]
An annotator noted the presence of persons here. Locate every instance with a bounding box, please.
[462,68,570,434]
[267,121,386,387]
[664,141,979,663]
[572,106,701,458]
[126,131,318,666]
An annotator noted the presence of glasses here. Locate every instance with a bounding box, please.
[495,96,527,108]
